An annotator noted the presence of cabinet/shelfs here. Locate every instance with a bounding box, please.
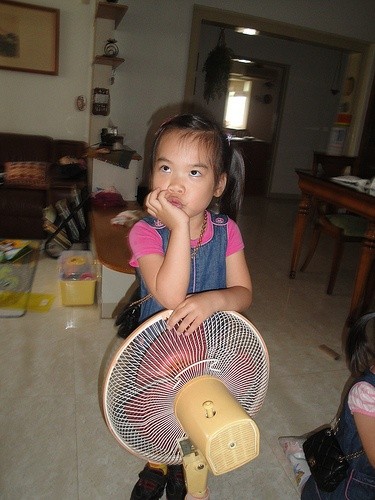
[93,3,129,68]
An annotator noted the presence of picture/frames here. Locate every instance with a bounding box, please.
[0,0,60,75]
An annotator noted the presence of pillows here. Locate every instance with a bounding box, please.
[3,161,51,190]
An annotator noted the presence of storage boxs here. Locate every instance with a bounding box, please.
[58,250,98,306]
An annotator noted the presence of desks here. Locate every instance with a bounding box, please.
[85,145,143,202]
[288,169,375,314]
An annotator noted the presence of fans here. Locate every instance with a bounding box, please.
[102,310,271,500]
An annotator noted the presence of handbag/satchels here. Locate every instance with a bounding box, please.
[115,293,141,340]
[302,427,351,493]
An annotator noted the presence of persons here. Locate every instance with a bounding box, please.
[128,114,251,499]
[300,311,375,500]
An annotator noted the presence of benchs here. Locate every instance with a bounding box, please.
[88,201,143,320]
[0,132,86,240]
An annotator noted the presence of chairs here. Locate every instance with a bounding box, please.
[299,151,368,295]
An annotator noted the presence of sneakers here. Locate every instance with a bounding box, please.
[130,462,167,500]
[166,465,188,500]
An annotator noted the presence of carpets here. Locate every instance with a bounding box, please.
[0,239,44,317]
